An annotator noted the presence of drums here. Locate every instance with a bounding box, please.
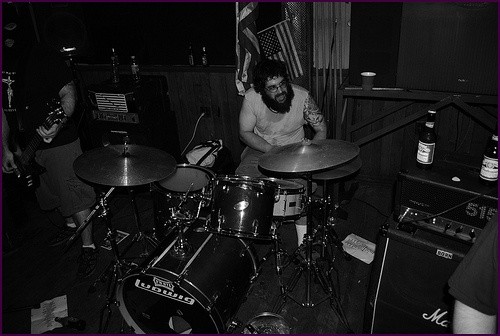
[113,229,261,336]
[205,174,280,242]
[258,176,306,220]
[153,164,216,232]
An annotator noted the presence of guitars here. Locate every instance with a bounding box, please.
[0,102,67,199]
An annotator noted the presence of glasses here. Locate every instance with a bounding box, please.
[265,81,286,93]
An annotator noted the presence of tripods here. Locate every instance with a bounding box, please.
[269,173,355,329]
[65,184,139,303]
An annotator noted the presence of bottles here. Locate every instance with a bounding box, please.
[111,48,120,73]
[478,132,499,192]
[201,46,208,66]
[415,109,437,170]
[130,55,141,81]
[187,41,196,66]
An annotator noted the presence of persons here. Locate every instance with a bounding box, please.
[2,6,99,266]
[449,215,497,334]
[236,60,328,227]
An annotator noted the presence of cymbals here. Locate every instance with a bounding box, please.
[257,139,360,172]
[72,142,178,187]
[301,157,363,179]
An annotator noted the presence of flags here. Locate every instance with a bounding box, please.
[256,21,303,81]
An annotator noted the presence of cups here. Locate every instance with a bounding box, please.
[361,72,376,91]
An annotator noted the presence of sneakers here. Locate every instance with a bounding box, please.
[46,224,78,246]
[75,246,99,279]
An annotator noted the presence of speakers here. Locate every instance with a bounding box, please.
[364,216,475,334]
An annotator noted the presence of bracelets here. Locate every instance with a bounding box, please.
[61,121,65,126]
